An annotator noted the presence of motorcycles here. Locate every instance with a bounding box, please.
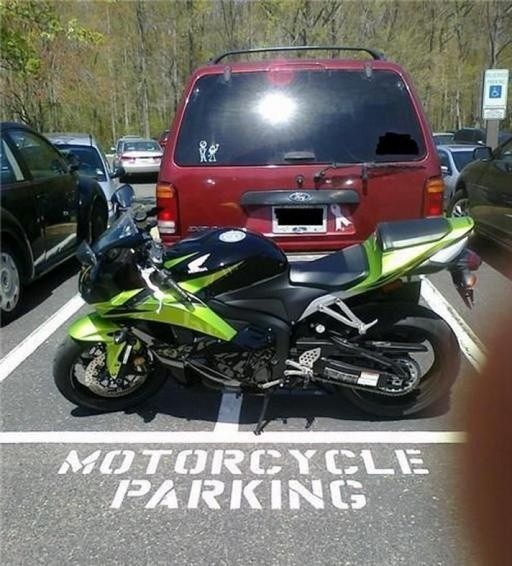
[45,162,473,431]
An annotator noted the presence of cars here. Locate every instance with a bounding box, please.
[0,120,108,320]
[446,131,512,253]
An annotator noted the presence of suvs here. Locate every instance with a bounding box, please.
[150,43,483,310]
